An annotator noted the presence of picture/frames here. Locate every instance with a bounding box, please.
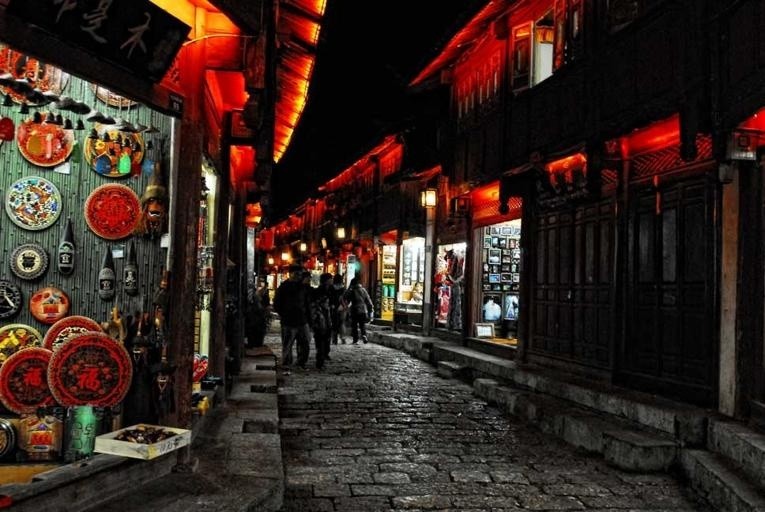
[473,323,496,339]
[483,226,521,321]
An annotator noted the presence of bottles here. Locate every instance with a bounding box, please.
[58,216,139,301]
[62,406,99,463]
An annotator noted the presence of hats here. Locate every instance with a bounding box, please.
[288,266,302,271]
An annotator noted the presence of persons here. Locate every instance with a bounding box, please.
[273,264,311,376]
[296,271,312,358]
[412,283,423,303]
[256,277,270,313]
[312,273,338,372]
[343,277,373,345]
[329,273,346,345]
